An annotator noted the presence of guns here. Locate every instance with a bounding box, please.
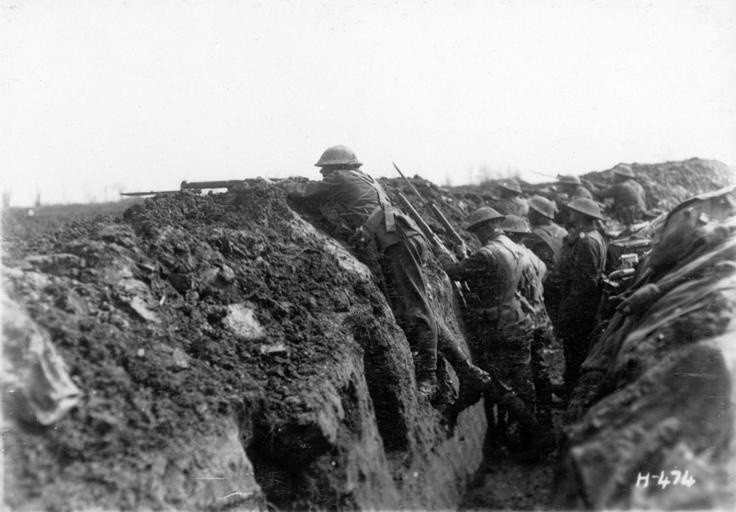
[556,173,606,188]
[120,177,301,197]
[392,163,539,316]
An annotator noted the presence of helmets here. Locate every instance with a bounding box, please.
[565,196,607,222]
[314,143,363,167]
[558,174,582,185]
[495,177,523,194]
[501,214,532,235]
[612,164,636,179]
[464,206,506,232]
[527,193,556,221]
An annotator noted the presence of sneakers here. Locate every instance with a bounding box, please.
[448,367,494,416]
[414,374,443,405]
